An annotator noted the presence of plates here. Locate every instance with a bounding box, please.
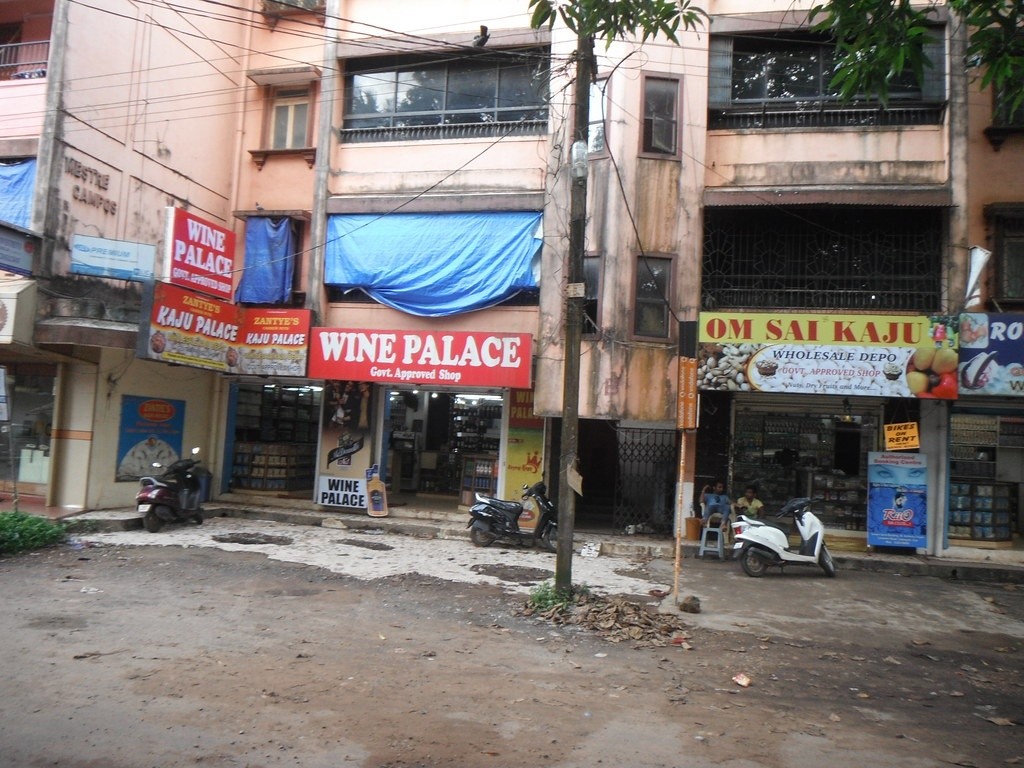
[744,344,776,391]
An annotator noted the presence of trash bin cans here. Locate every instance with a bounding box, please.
[192,473,214,503]
[685,518,702,541]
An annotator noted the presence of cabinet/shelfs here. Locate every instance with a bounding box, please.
[384,395,504,503]
[948,413,1024,482]
[227,385,323,491]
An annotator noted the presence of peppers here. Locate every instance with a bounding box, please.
[928,374,958,398]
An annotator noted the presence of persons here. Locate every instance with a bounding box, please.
[736,486,765,521]
[699,481,748,532]
[323,380,372,433]
[868,464,928,484]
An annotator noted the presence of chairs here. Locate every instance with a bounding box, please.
[700,503,730,544]
[729,503,751,537]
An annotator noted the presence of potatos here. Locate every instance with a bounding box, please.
[905,345,958,393]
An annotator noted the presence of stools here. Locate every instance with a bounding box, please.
[698,529,726,562]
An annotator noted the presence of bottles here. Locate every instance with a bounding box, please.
[367,473,388,517]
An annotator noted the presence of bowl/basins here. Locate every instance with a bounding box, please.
[756,361,779,375]
[883,369,902,380]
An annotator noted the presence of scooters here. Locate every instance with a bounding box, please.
[134,446,204,533]
[466,469,560,553]
[730,495,836,578]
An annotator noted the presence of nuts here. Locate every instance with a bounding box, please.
[698,344,765,392]
